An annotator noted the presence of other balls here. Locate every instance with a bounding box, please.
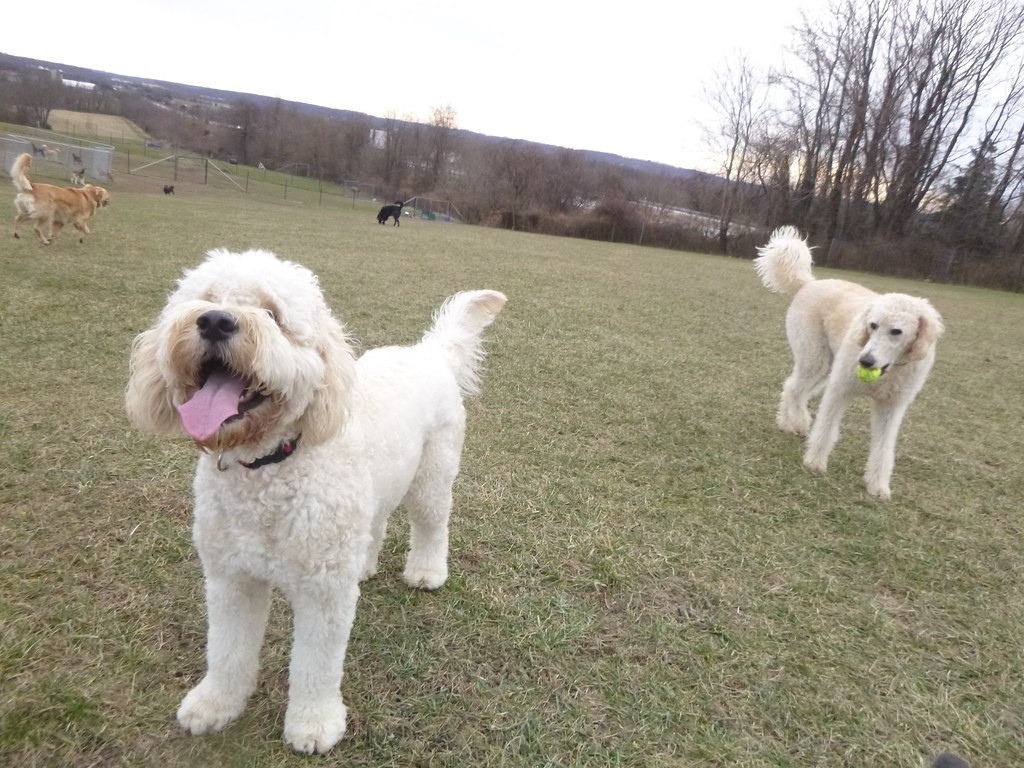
[856,363,882,382]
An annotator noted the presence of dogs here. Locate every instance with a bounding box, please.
[164,185,175,195]
[8,153,112,245]
[751,225,943,500]
[125,247,510,751]
[377,200,403,226]
[31,142,61,162]
